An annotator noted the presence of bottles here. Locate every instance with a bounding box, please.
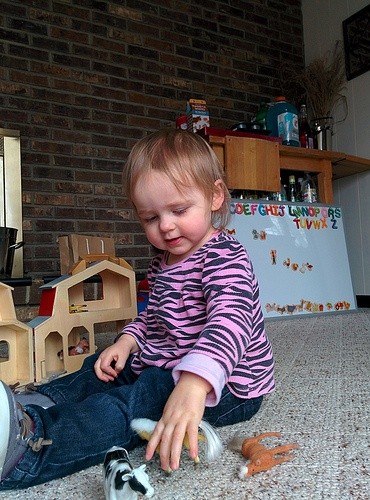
[253,97,308,147]
[271,174,318,203]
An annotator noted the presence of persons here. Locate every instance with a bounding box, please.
[0,129,277,491]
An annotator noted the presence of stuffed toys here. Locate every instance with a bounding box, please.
[130,418,223,474]
[229,430,299,479]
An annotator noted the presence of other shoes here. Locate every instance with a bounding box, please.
[0,380,54,482]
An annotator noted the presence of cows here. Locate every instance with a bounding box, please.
[102,446,154,500]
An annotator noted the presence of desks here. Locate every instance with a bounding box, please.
[204,134,370,204]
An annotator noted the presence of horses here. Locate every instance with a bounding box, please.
[131,418,224,476]
[227,430,299,481]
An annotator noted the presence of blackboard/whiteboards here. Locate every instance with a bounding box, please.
[218,201,361,322]
